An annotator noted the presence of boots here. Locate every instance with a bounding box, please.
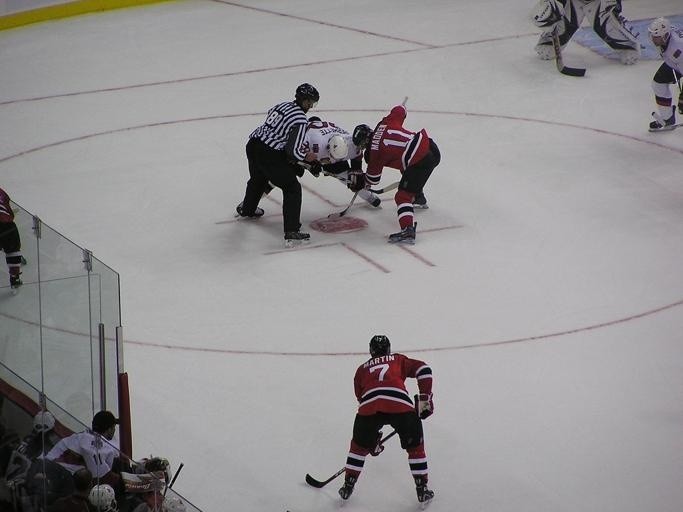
[337,483,354,499]
[411,192,428,206]
[647,105,677,133]
[387,220,417,241]
[9,271,25,289]
[415,484,435,502]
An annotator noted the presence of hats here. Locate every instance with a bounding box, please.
[31,410,57,433]
[91,409,121,434]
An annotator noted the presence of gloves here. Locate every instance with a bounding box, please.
[418,392,434,420]
[369,430,386,457]
[346,169,367,193]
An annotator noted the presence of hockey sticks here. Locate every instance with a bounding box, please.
[306,405,429,488]
[300,160,400,194]
[652,112,682,130]
[555,25,585,77]
[329,96,406,220]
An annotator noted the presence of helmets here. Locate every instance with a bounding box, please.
[647,16,671,37]
[144,456,171,475]
[327,134,349,160]
[352,123,369,147]
[368,334,392,355]
[87,482,117,509]
[295,82,320,101]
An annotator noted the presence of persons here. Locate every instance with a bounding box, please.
[526,0,641,65]
[346,106,441,239]
[0,188,23,289]
[648,16,683,128]
[338,335,434,502]
[236,117,381,218]
[243,83,320,240]
[0,410,186,512]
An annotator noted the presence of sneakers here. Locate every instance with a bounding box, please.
[233,205,265,222]
[283,228,311,241]
[365,191,382,208]
